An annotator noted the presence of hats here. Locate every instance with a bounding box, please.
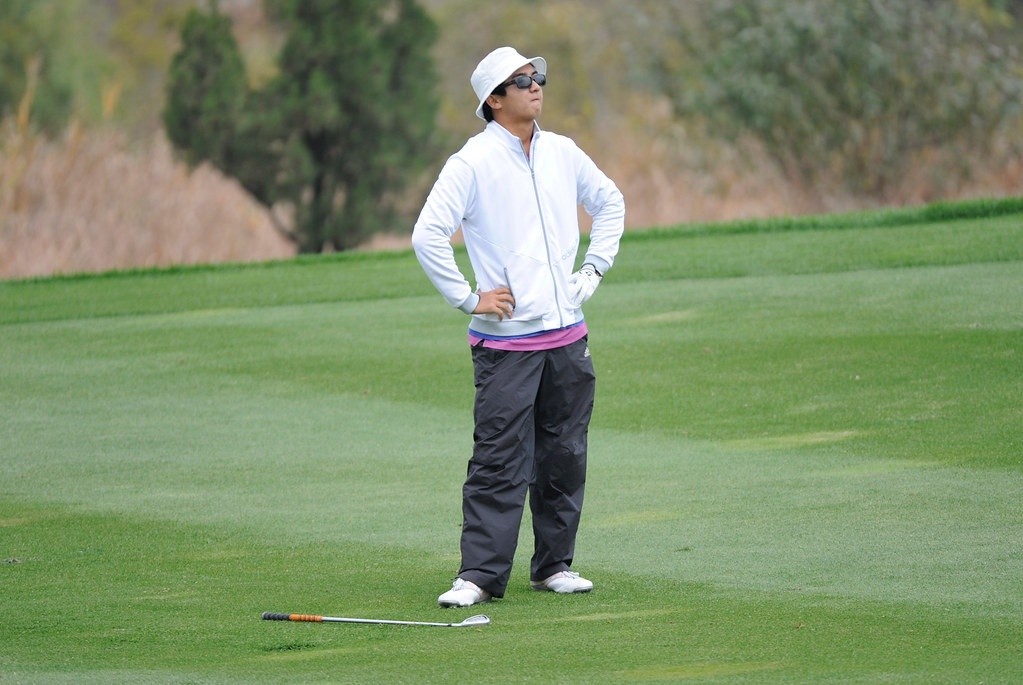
[470,46,547,121]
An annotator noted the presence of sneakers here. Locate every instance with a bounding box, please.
[439,577,492,608]
[533,569,594,595]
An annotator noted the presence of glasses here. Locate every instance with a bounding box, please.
[502,74,547,89]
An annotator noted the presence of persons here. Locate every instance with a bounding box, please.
[412,47,626,605]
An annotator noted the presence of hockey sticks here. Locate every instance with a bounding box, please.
[260,610,490,627]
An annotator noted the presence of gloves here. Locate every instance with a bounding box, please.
[567,264,603,306]
